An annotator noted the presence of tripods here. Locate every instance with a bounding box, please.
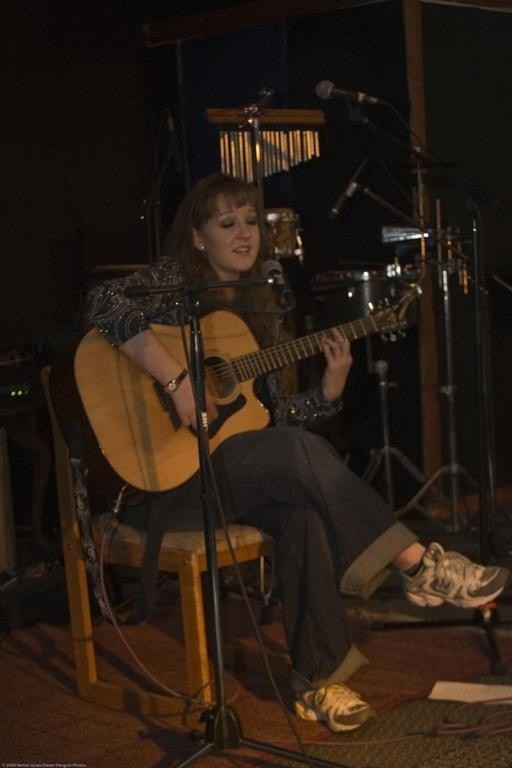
[362,359,443,519]
[394,268,510,536]
[368,211,512,678]
[153,317,353,768]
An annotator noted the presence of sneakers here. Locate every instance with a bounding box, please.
[289,684,379,739]
[398,542,511,617]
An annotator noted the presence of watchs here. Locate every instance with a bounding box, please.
[164,369,187,396]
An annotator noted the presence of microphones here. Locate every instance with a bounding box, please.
[236,85,278,129]
[347,110,427,161]
[332,179,359,216]
[315,80,383,108]
[261,259,295,308]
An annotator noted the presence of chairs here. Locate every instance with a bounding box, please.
[40,362,278,709]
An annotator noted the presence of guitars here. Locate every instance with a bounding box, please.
[65,297,409,508]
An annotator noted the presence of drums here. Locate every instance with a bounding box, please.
[309,268,412,336]
[76,265,149,338]
[263,207,303,260]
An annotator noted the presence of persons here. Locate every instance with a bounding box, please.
[84,171,510,736]
